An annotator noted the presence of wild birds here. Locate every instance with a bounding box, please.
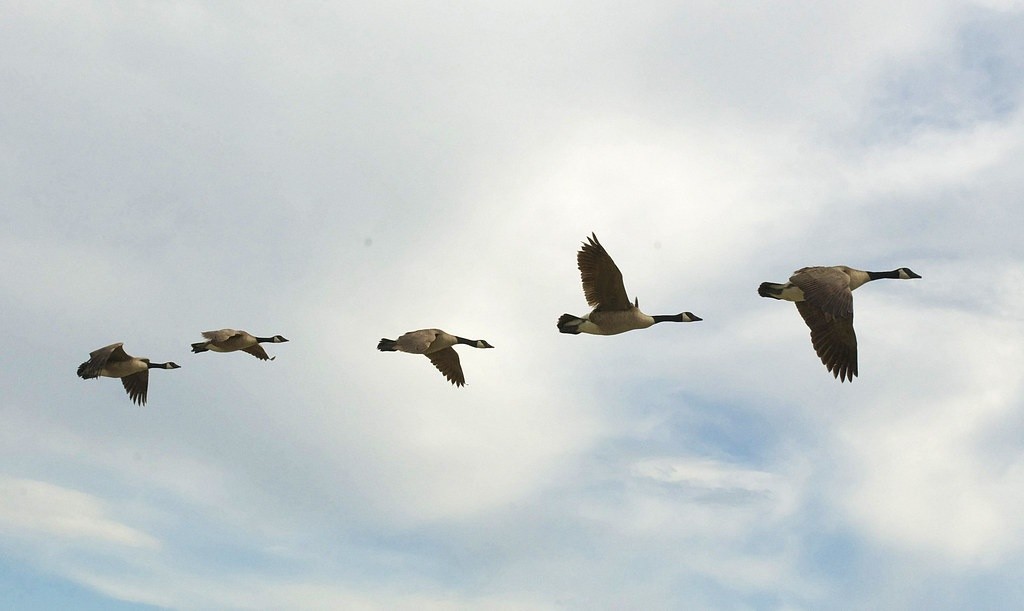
[757,265,923,385]
[376,328,495,389]
[557,231,704,336]
[190,328,291,362]
[76,342,182,407]
